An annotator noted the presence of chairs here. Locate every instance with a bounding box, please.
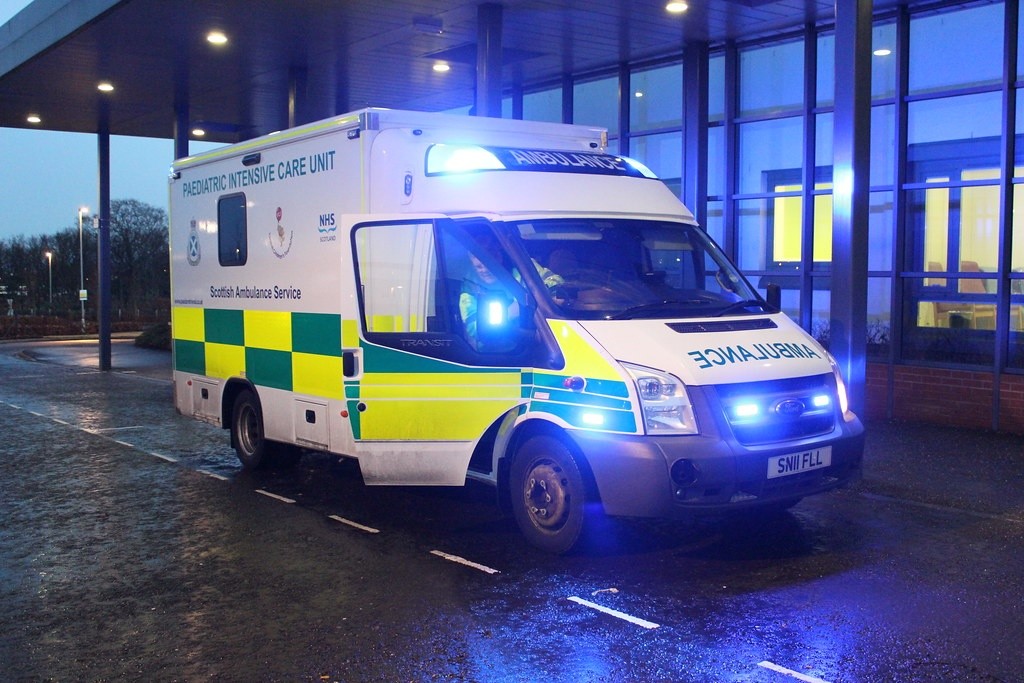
[925,261,1024,331]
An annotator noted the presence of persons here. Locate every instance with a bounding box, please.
[456,240,573,357]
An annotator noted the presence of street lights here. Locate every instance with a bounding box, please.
[77,207,88,332]
[45,252,52,314]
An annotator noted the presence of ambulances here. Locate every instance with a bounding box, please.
[165,107,864,560]
